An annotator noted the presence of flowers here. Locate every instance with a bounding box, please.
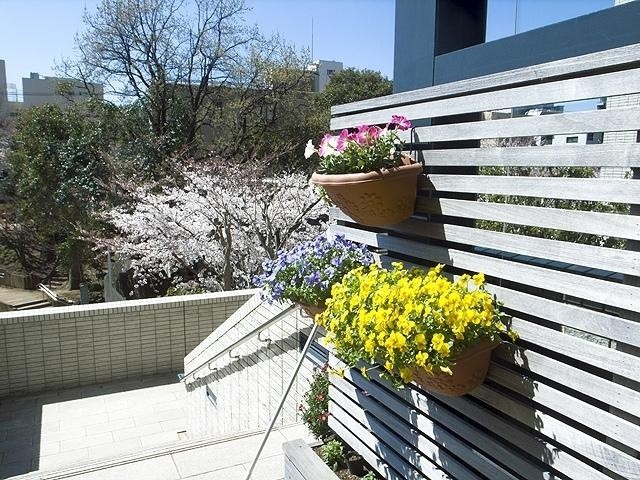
[315,261,524,386]
[303,115,413,177]
[254,232,375,308]
[298,362,332,442]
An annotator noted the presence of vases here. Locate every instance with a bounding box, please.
[308,162,423,229]
[412,336,500,394]
[295,298,326,321]
